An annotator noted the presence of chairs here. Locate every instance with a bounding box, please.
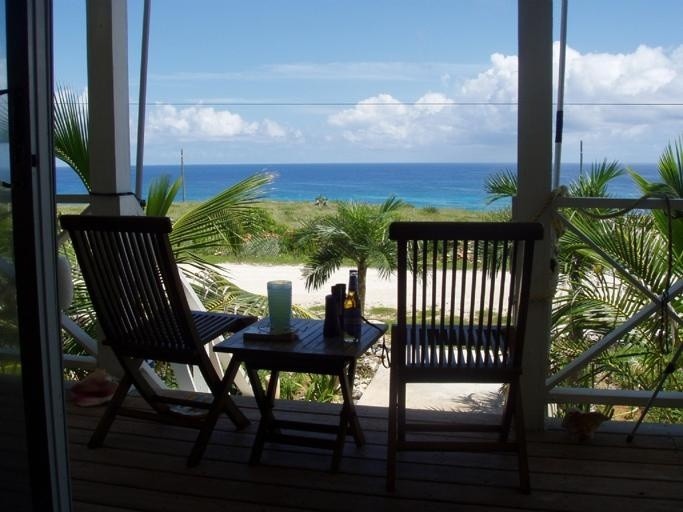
[59,215,258,468]
[385,222,544,492]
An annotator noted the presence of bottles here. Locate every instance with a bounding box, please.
[323,269,361,346]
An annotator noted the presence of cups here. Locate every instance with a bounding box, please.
[266,280,292,330]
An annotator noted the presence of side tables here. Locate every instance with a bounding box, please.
[213,319,388,472]
